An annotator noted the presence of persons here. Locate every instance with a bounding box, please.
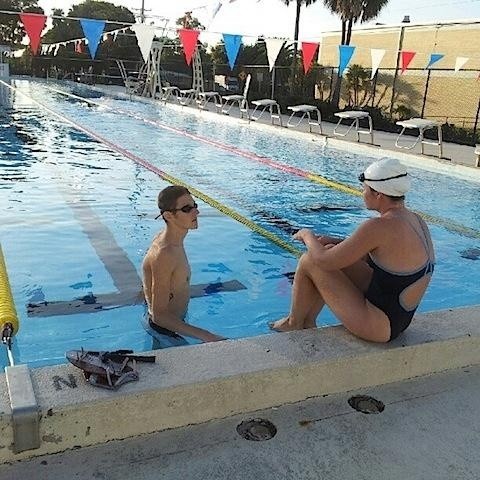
[268,157,435,344]
[141,186,227,350]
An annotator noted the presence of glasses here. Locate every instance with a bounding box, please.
[358,173,407,182]
[165,202,198,213]
